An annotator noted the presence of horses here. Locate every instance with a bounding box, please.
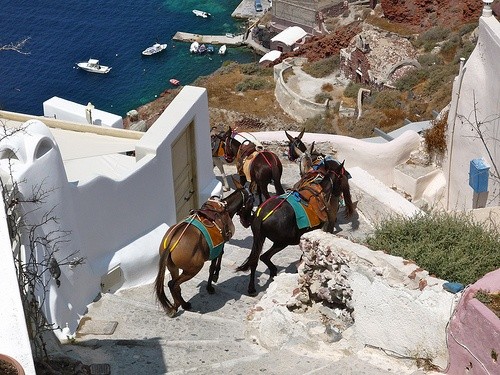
[233,159,346,297]
[152,173,256,313]
[215,126,284,205]
[284,127,354,235]
[210,125,231,192]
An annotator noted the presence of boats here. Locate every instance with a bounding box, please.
[217,44,226,55]
[142,42,167,55]
[189,41,214,54]
[75,58,112,74]
[169,79,180,86]
[192,9,211,18]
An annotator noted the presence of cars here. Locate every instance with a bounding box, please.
[255,0,263,12]
